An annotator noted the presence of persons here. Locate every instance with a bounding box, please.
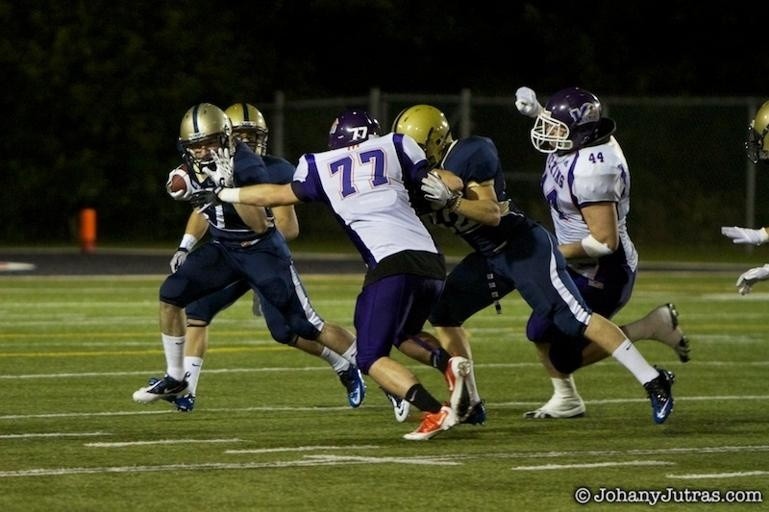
[132,103,414,425]
[719,104,769,295]
[512,84,691,420]
[147,103,369,414]
[190,105,475,443]
[389,100,679,431]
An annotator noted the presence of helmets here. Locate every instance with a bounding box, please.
[392,105,452,169]
[746,101,769,164]
[225,103,269,156]
[327,111,382,152]
[178,102,232,174]
[540,87,603,150]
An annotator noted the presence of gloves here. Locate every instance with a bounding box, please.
[515,87,542,118]
[169,247,189,273]
[202,148,237,188]
[735,263,769,295]
[720,225,768,246]
[189,187,223,205]
[421,171,461,212]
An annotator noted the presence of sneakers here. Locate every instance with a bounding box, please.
[379,355,486,441]
[336,362,365,408]
[643,365,675,423]
[132,372,195,411]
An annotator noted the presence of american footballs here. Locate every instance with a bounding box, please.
[171,165,191,193]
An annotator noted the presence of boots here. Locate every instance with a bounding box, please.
[523,386,587,419]
[637,302,690,362]
